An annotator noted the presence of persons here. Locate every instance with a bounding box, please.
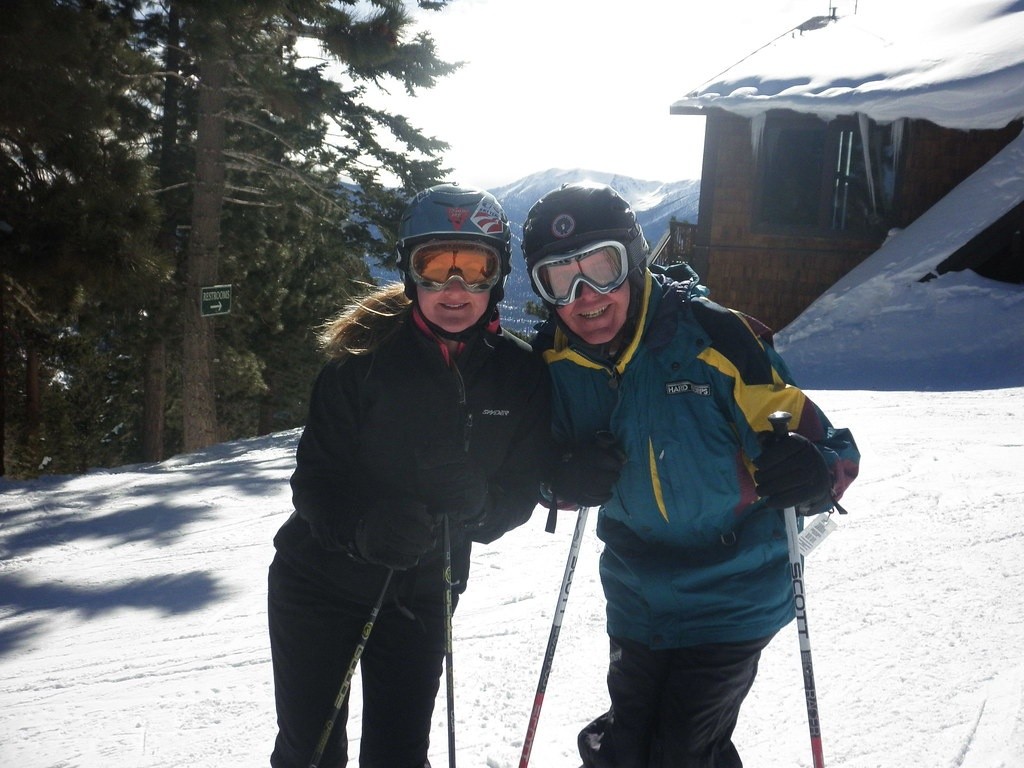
[268,183,553,768]
[520,187,860,768]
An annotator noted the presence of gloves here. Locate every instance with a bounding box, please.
[753,430,848,514]
[346,486,445,570]
[417,434,499,531]
[534,430,626,534]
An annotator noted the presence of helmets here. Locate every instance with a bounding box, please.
[520,183,647,311]
[396,182,512,277]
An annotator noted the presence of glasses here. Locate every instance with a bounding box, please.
[532,239,628,305]
[408,240,501,293]
